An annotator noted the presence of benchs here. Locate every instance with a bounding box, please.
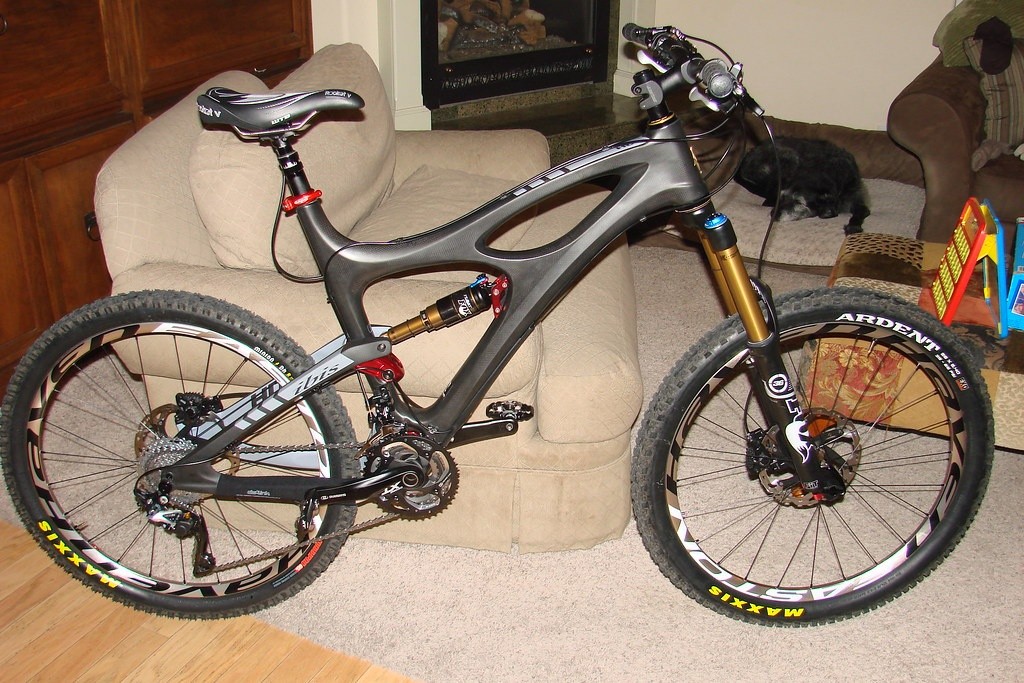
[793,231,1023,452]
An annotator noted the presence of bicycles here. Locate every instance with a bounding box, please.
[0,24,995,627]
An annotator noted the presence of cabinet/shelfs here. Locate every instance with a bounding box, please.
[0,0,315,409]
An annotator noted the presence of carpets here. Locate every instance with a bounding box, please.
[654,170,927,267]
[0,241,1021,683]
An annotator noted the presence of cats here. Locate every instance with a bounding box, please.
[738,137,871,234]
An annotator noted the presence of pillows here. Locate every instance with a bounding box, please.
[932,0,1022,77]
[963,35,1023,172]
[187,39,398,282]
[349,165,539,283]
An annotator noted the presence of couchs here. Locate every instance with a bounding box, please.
[93,34,642,554]
[886,49,1024,245]
